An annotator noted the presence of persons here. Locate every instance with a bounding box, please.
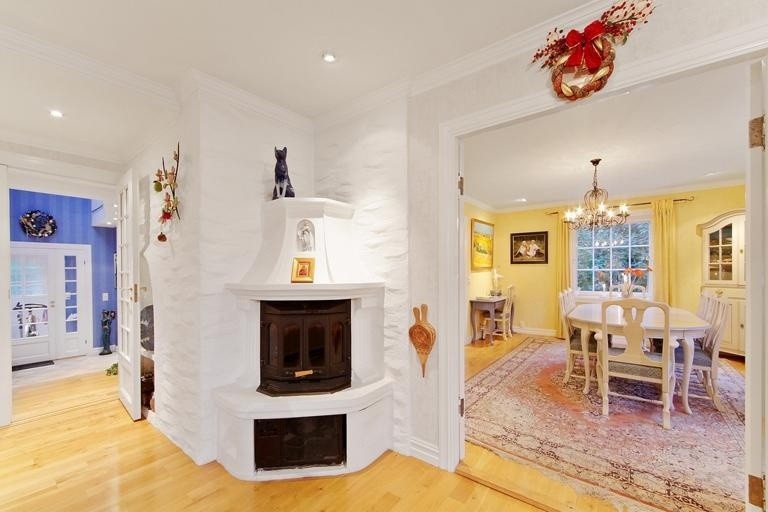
[99,310,113,356]
[301,220,313,252]
[527,239,544,259]
[513,240,528,257]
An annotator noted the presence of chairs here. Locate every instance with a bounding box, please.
[480,284,515,342]
[559,284,729,430]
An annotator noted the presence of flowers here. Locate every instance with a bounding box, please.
[153,141,180,224]
[18,210,57,238]
[623,256,654,292]
[531,0,656,70]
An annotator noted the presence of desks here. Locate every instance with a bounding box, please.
[469,297,514,344]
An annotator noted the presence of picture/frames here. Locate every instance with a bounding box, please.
[471,218,495,272]
[291,257,315,282]
[511,231,548,264]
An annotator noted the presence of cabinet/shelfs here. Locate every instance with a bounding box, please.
[696,209,745,357]
[10,241,93,367]
[257,300,351,397]
[254,416,346,471]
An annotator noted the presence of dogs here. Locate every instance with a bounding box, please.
[272,145,295,200]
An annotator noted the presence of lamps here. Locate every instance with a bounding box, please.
[562,158,630,231]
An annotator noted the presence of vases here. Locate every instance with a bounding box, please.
[34,237,40,242]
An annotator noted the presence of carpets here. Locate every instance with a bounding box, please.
[465,337,745,512]
